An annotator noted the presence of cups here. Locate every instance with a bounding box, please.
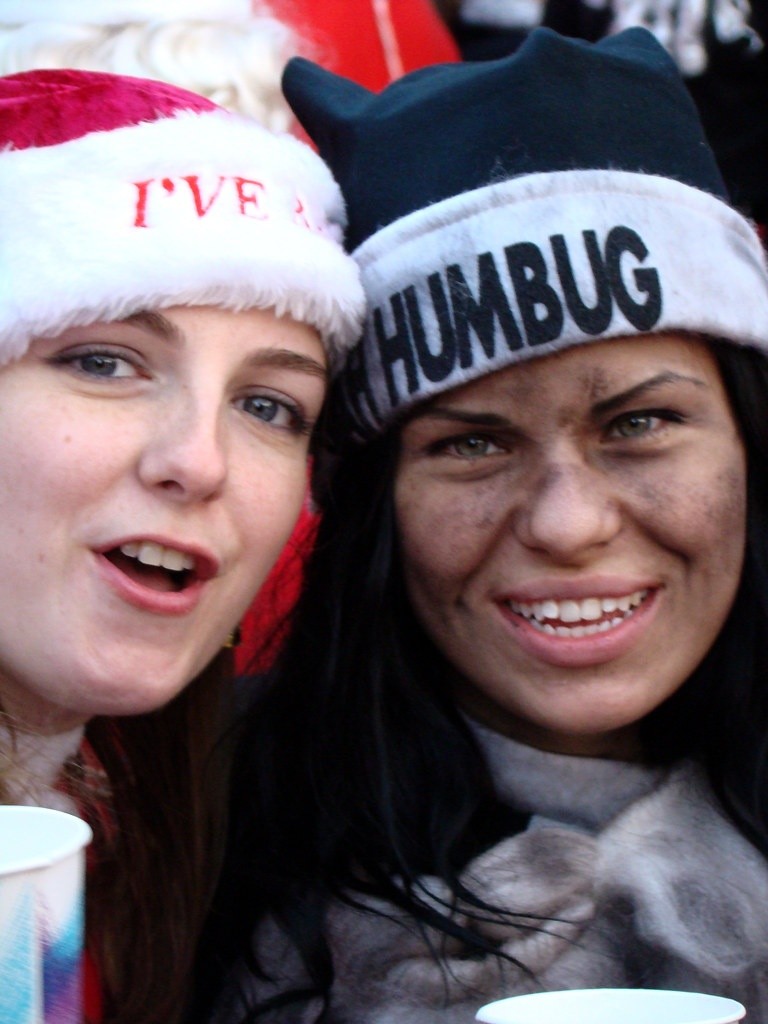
[474,987,749,1024]
[0,801,98,1024]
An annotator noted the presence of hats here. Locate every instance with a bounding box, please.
[278,28,768,435]
[1,70,368,381]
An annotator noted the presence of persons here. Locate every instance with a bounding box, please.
[0,0,768,1024]
